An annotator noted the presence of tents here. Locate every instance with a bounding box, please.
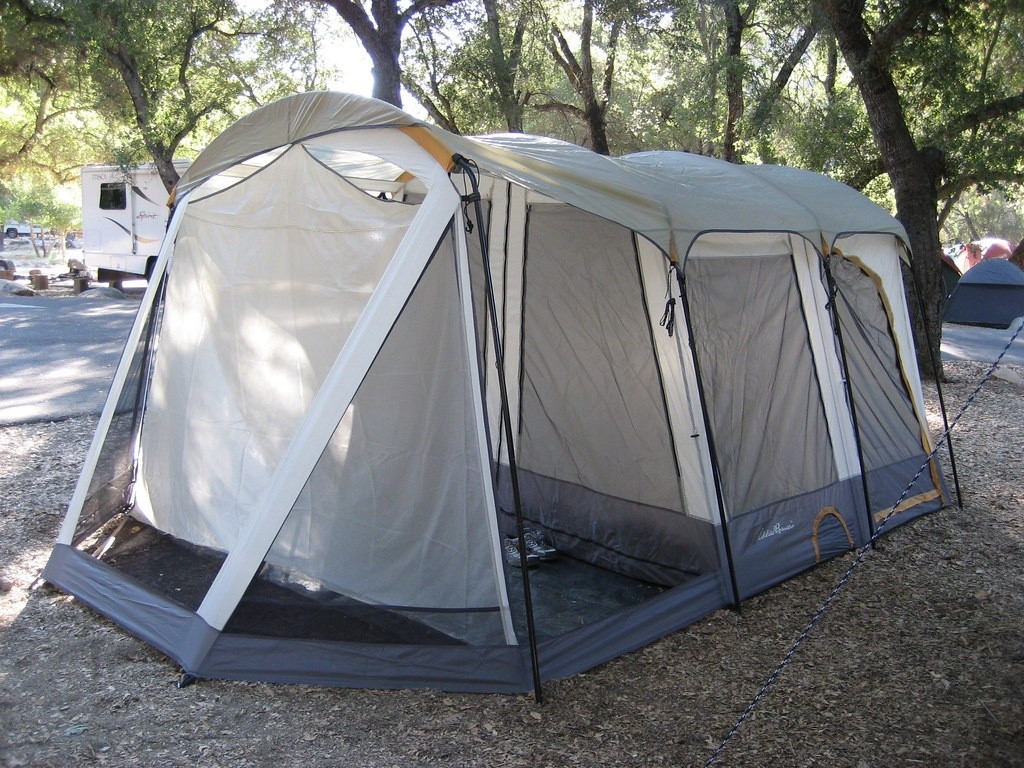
[40,93,953,693]
[938,238,1024,330]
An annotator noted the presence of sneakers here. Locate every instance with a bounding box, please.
[504,537,540,567]
[523,528,558,560]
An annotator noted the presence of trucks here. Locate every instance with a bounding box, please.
[81,159,197,293]
[3,219,48,238]
